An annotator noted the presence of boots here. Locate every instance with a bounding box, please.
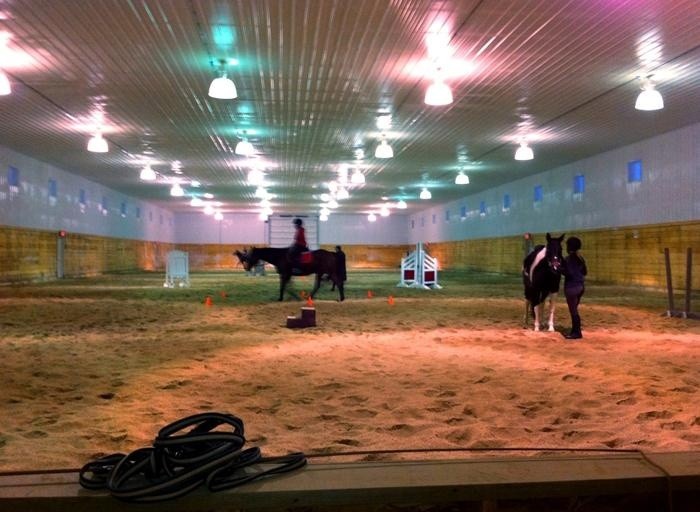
[566,314,583,339]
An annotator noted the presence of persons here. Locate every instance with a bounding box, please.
[329,245,346,291]
[560,236,588,339]
[523,232,534,258]
[285,219,309,272]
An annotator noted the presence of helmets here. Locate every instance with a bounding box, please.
[293,219,303,224]
[566,237,581,249]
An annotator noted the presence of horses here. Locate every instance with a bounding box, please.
[523,232,566,333]
[231,249,246,268]
[242,246,346,301]
